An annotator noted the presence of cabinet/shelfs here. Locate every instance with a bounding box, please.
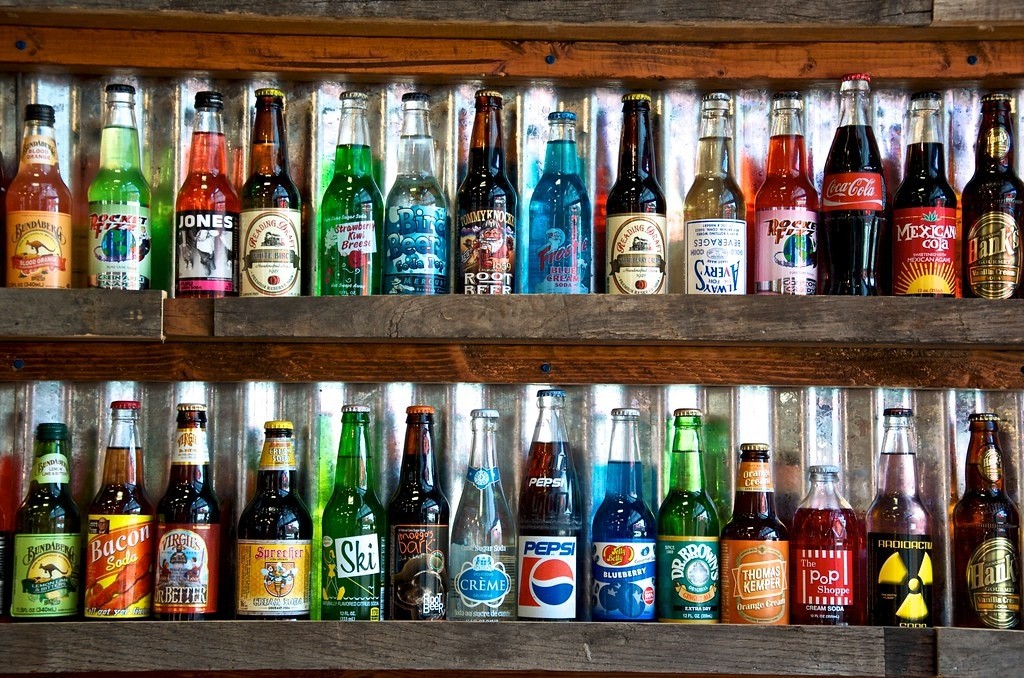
[0,0,1024,678]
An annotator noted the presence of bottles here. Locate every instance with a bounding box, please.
[794,466,861,629]
[177,91,239,297]
[0,389,657,622]
[962,92,1024,299]
[604,92,667,295]
[755,92,822,297]
[889,92,957,298]
[239,87,303,298]
[681,94,749,295]
[866,407,933,627]
[4,104,73,291]
[528,112,590,294]
[455,89,517,297]
[658,409,719,625]
[85,84,152,292]
[317,89,383,297]
[718,443,788,625]
[821,73,890,296]
[953,413,1024,631]
[384,92,452,296]
